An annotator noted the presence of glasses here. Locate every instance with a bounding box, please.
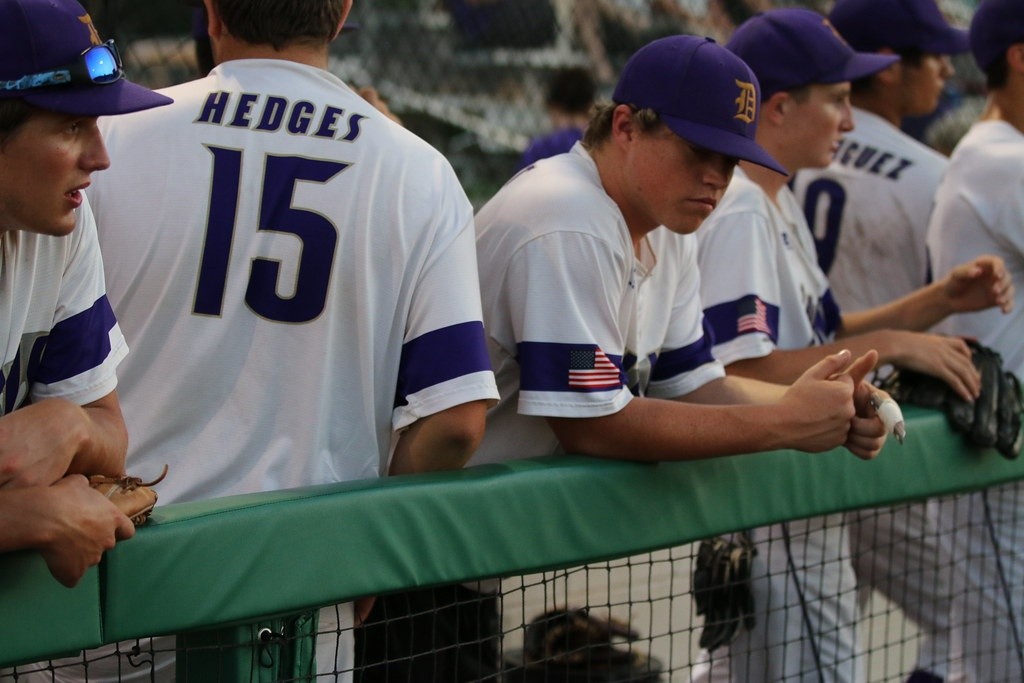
[1,37,125,99]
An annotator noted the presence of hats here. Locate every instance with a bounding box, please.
[1,0,174,116]
[828,0,971,54]
[968,0,1024,49]
[724,8,901,83]
[612,35,791,178]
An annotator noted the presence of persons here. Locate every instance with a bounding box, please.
[688,11,1015,683]
[924,1,1023,683]
[553,0,714,102]
[520,65,597,168]
[1,0,500,682]
[354,31,905,682]
[907,55,991,151]
[794,0,976,683]
[0,0,173,589]
